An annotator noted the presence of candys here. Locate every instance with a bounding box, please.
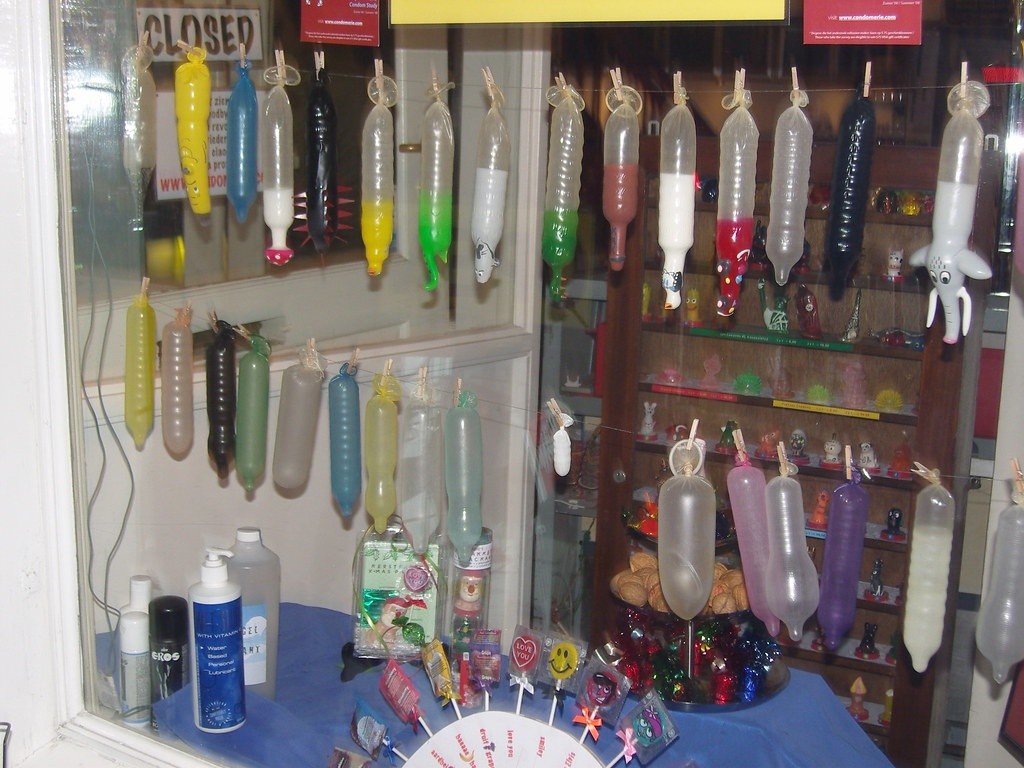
[330,628,667,768]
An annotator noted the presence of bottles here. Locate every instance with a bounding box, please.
[188,547,247,734]
[229,528,281,699]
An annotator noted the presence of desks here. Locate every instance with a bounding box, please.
[95,602,895,768]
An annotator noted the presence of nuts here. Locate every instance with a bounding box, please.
[609,551,749,617]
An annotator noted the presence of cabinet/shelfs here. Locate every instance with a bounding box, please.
[584,127,1002,768]
[530,278,607,632]
